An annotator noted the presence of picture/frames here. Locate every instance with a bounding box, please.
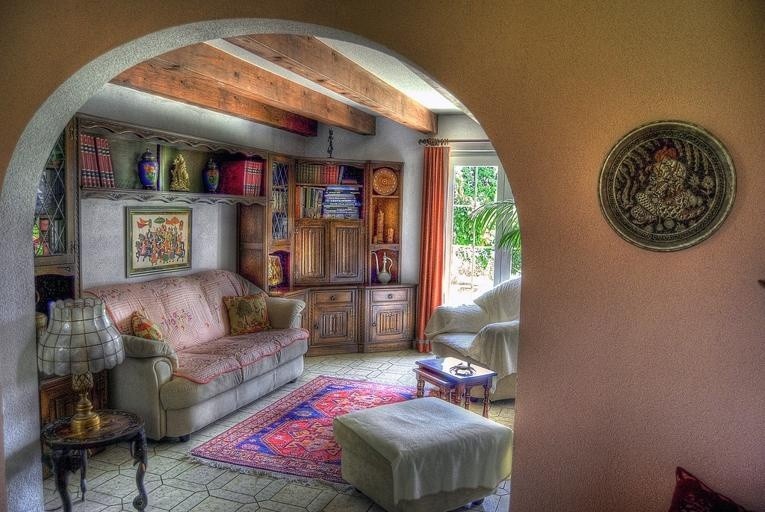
[123,205,193,279]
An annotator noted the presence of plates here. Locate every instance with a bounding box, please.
[372,168,397,196]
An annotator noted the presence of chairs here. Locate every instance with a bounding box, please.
[431,275,521,403]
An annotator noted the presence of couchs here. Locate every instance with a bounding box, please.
[83,268,309,444]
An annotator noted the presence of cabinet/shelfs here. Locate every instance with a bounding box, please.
[74,111,268,209]
[235,149,419,358]
[32,115,110,480]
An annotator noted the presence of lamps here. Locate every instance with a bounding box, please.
[37,297,126,434]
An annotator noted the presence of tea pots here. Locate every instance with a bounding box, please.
[371,251,394,286]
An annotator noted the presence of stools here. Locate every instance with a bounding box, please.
[332,396,513,512]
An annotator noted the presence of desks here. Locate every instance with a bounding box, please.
[40,408,148,511]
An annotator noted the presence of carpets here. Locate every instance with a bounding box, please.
[184,373,464,497]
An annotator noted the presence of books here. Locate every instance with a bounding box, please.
[80,134,117,188]
[220,160,264,197]
[32,216,65,255]
[296,165,362,219]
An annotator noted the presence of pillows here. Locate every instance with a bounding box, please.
[130,310,180,374]
[221,291,272,338]
